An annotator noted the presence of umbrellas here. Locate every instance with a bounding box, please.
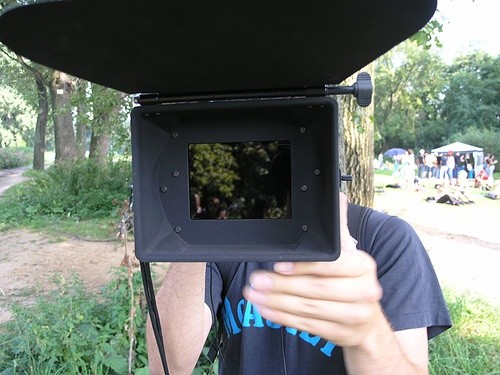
[384,148,407,156]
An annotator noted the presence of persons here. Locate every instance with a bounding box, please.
[378,149,500,205]
[146,191,452,375]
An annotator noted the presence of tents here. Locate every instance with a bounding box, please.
[430,142,483,155]
